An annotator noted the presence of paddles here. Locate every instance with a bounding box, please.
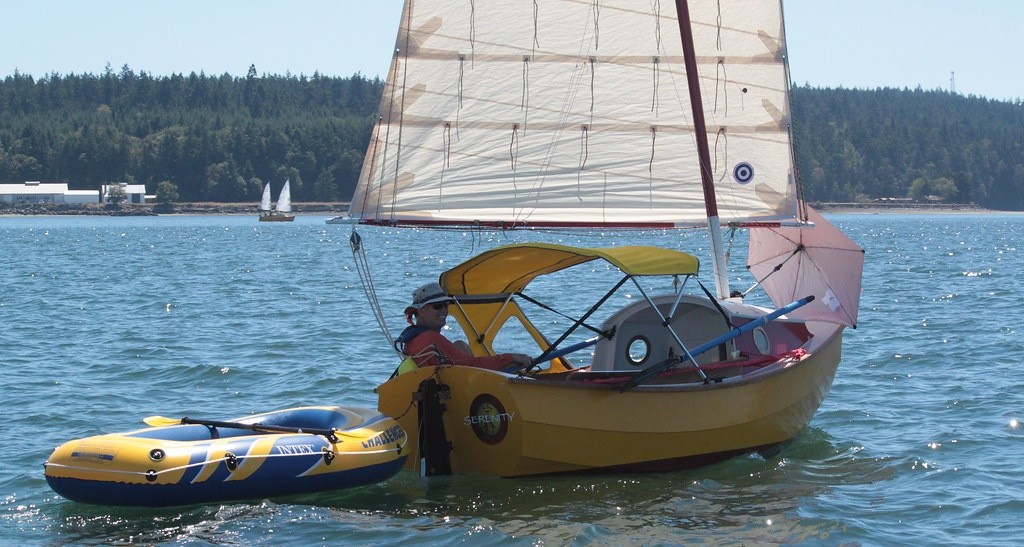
[504,336,598,373]
[142,415,371,440]
[619,295,815,394]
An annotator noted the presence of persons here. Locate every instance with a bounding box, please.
[259,211,273,218]
[402,283,532,370]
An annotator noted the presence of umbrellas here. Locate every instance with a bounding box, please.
[741,200,865,330]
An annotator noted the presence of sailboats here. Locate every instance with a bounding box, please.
[333,0,867,473]
[258,178,296,222]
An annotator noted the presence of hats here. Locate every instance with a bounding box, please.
[410,282,454,308]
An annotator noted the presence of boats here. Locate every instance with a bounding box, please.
[42,402,411,510]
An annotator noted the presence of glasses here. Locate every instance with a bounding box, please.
[432,302,448,310]
